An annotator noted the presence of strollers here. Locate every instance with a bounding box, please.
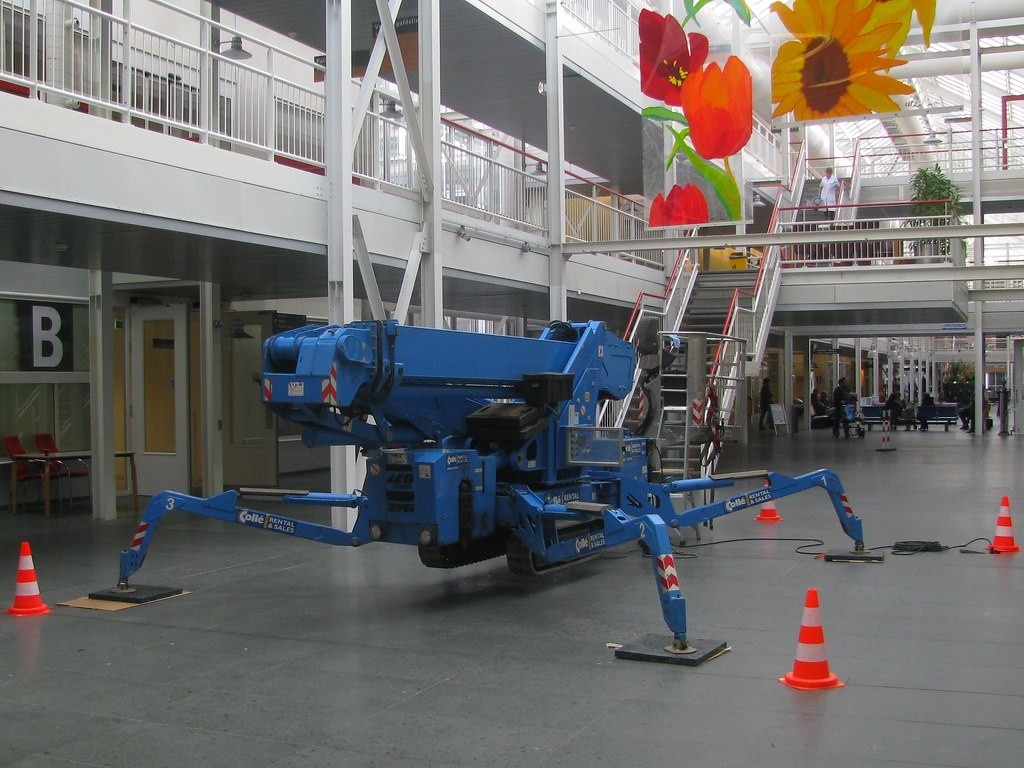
[838,402,865,438]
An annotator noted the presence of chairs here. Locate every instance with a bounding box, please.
[5,434,62,510]
[33,432,93,511]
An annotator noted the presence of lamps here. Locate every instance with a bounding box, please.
[753,195,766,207]
[624,202,640,213]
[215,318,253,340]
[379,100,404,119]
[923,130,942,145]
[523,161,546,176]
[216,35,253,61]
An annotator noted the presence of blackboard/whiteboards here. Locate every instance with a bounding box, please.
[770,404,787,425]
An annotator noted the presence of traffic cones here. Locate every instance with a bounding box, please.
[775,586,847,691]
[984,495,1023,552]
[2,541,53,617]
[753,480,785,522]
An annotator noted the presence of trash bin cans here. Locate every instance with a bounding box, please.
[728,251,748,270]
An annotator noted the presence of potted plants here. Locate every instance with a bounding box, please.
[902,164,968,264]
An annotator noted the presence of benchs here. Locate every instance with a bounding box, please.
[861,402,959,432]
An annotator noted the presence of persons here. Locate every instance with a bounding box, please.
[959,392,975,433]
[832,377,857,438]
[818,167,840,219]
[758,378,775,430]
[918,393,931,430]
[884,392,906,430]
[811,388,834,416]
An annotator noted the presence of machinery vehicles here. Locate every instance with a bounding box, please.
[79,317,885,670]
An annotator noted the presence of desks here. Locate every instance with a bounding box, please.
[14,450,138,517]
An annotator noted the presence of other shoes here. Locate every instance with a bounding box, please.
[967,430,974,433]
[769,426,774,429]
[960,426,968,429]
[759,426,766,429]
